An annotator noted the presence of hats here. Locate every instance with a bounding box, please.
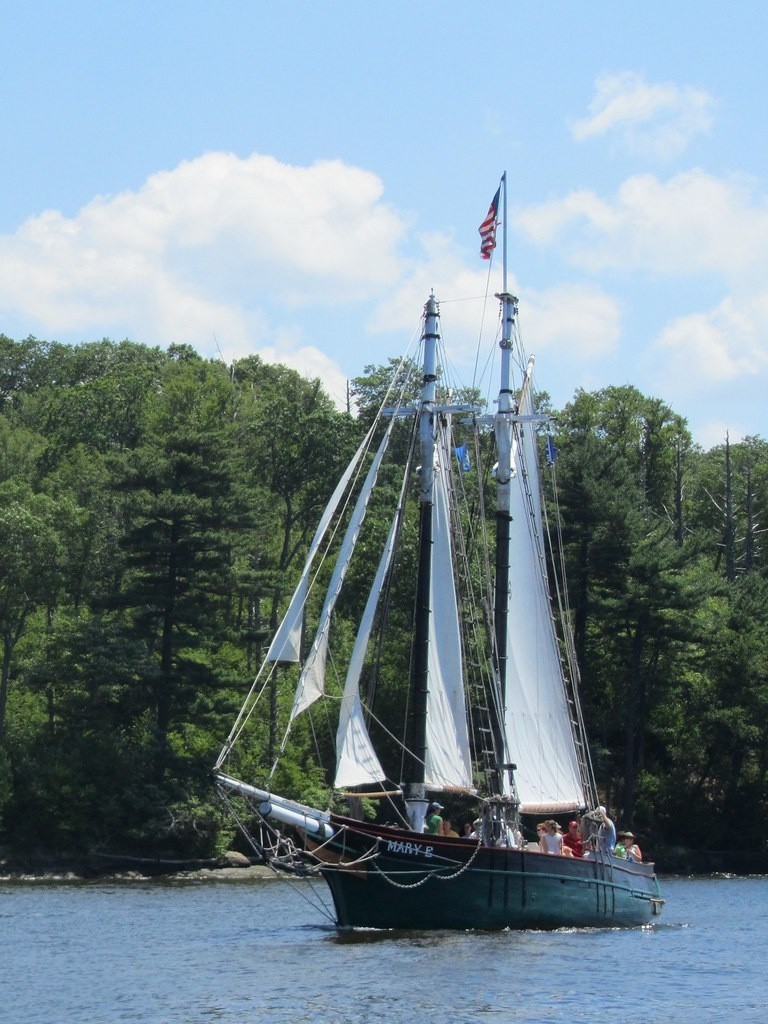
[545,819,561,829]
[431,802,444,810]
[569,821,578,828]
[473,818,483,828]
[625,832,634,837]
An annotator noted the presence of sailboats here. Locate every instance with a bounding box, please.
[206,169,666,932]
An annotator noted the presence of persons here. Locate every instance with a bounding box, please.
[462,822,472,839]
[541,820,562,854]
[443,818,459,838]
[615,831,626,858]
[422,801,444,836]
[537,824,547,839]
[562,821,585,858]
[469,818,483,839]
[623,832,642,862]
[595,806,616,855]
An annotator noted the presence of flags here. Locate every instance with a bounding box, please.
[453,443,470,472]
[478,176,501,259]
[544,437,559,467]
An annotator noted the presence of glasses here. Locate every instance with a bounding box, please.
[536,829,542,831]
[629,837,633,839]
